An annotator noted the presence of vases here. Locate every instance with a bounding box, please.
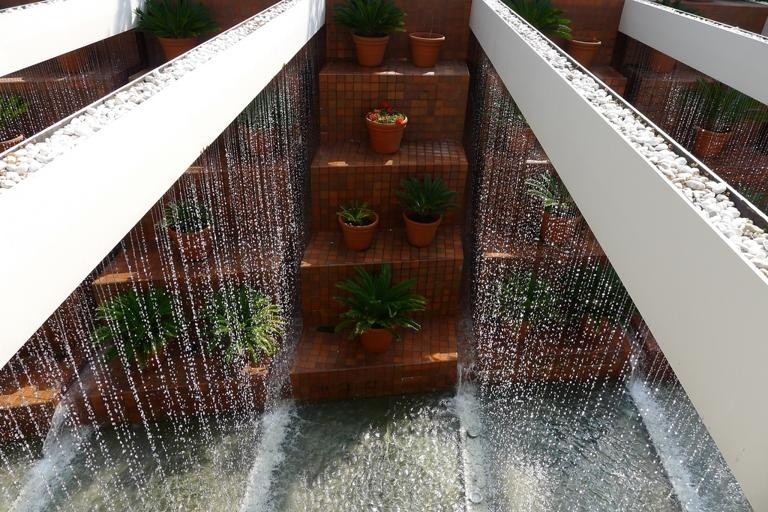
[566,36,602,70]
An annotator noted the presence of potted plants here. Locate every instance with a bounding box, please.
[391,170,459,248]
[330,2,405,66]
[675,76,768,158]
[366,99,410,157]
[410,28,448,69]
[133,0,219,63]
[334,202,380,252]
[88,189,289,378]
[494,166,637,350]
[0,94,35,150]
[329,257,432,354]
